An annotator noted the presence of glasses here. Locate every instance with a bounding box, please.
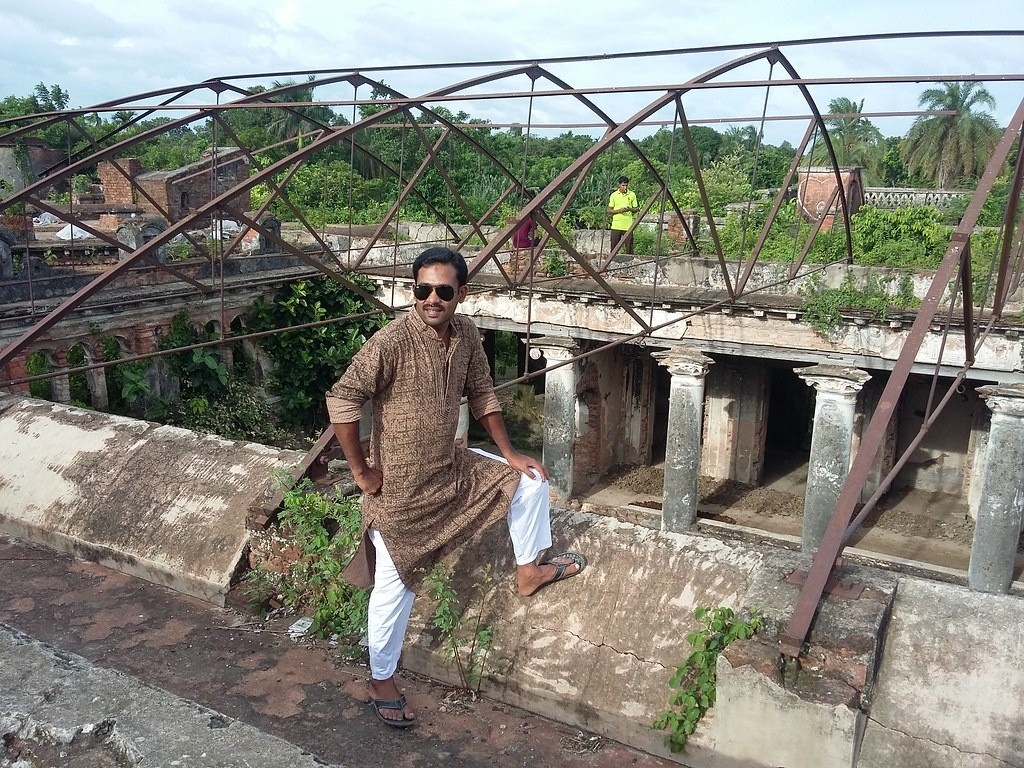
[412,284,462,302]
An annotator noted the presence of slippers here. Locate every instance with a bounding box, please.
[527,552,588,596]
[373,693,415,726]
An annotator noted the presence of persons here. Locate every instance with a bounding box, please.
[324,247,587,726]
[512,215,541,248]
[608,176,639,255]
